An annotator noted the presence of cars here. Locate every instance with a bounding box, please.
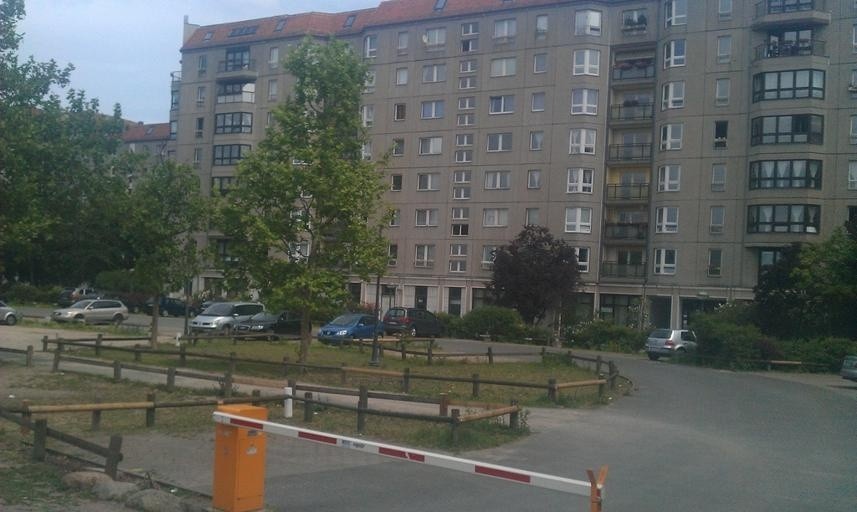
[840,355,857,382]
[644,327,700,361]
[0,299,21,326]
